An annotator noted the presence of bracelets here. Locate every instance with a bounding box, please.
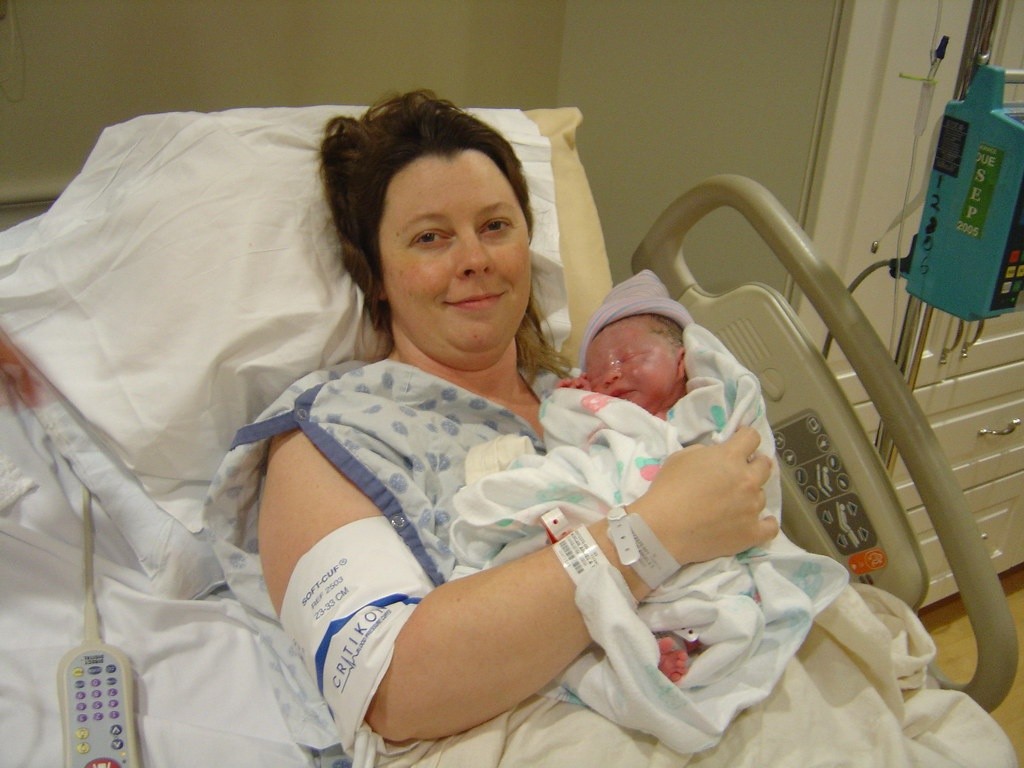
[540,508,681,614]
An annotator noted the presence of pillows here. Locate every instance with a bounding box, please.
[97,106,613,369]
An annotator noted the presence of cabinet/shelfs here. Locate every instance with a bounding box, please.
[783,0,1024,610]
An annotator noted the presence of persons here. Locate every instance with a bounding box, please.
[518,270,782,751]
[201,91,778,746]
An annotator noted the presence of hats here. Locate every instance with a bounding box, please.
[580,269,694,372]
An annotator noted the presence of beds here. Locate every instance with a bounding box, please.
[0,103,1024,768]
